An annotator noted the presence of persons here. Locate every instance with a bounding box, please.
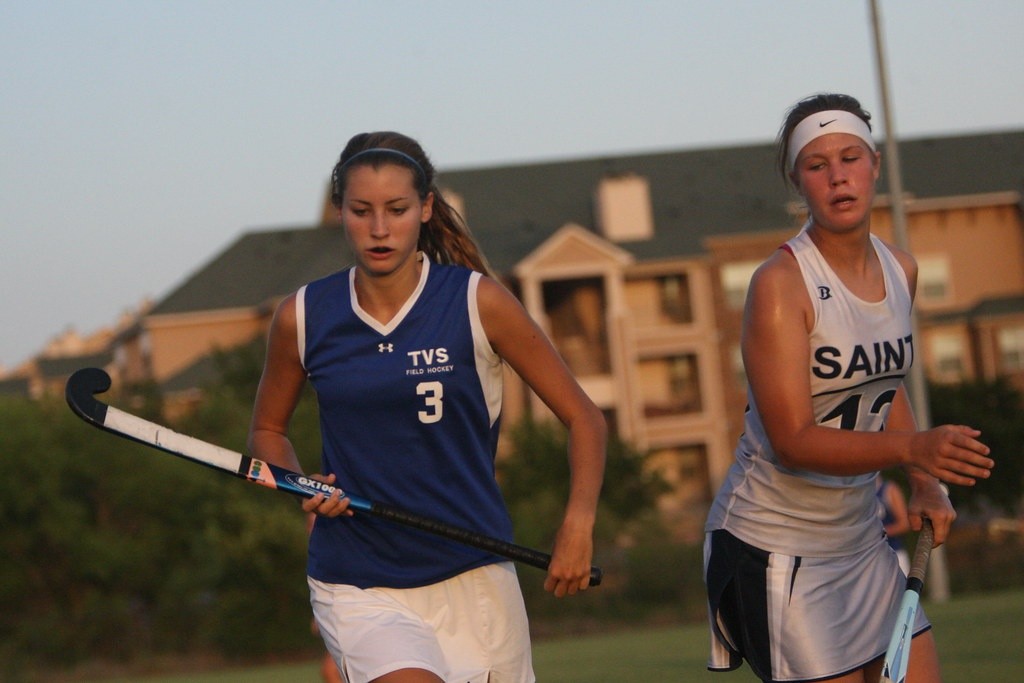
[874,475,910,578]
[703,93,995,683]
[247,131,610,683]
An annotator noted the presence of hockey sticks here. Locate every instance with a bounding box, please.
[65,366,601,588]
[879,481,951,683]
